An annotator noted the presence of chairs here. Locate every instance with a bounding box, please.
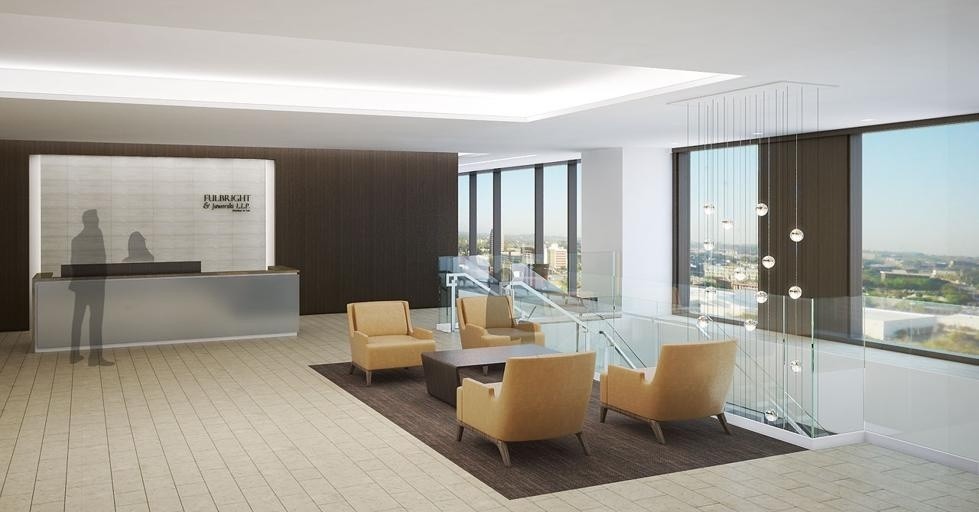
[455,352,597,467]
[455,294,545,349]
[346,300,437,387]
[600,339,737,445]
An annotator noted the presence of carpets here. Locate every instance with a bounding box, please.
[308,361,809,500]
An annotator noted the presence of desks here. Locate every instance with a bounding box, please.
[421,344,562,408]
